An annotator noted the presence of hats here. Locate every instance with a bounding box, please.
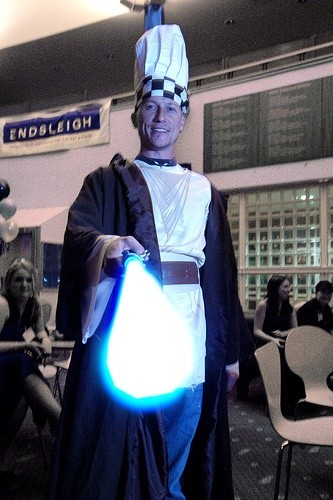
[134,24,189,115]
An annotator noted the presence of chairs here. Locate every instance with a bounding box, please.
[37,344,77,404]
[253,325,333,499]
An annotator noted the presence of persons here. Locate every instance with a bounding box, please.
[51,24,240,500]
[298,279,332,335]
[0,258,61,462]
[253,276,300,349]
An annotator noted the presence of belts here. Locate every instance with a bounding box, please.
[103,259,199,286]
